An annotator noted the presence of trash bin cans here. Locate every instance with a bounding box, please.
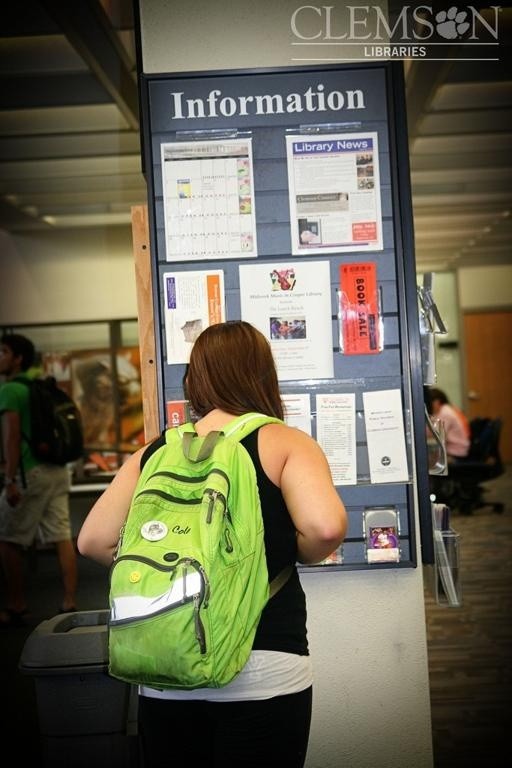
[17,608,133,739]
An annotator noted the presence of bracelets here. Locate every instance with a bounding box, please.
[3,476,17,486]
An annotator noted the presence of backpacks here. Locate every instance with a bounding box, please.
[107,410,295,691]
[8,375,85,466]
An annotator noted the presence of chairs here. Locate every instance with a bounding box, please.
[432,417,509,515]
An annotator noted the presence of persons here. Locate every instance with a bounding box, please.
[0,331,77,617]
[424,384,471,468]
[75,320,348,768]
